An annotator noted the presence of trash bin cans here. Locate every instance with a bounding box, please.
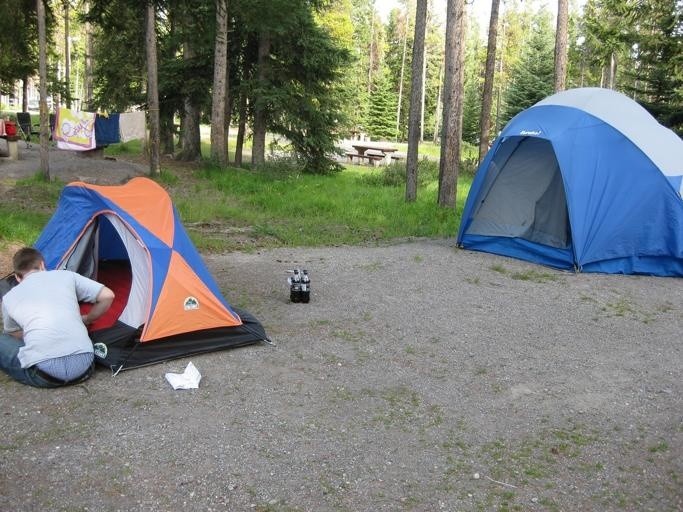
[364,135,370,142]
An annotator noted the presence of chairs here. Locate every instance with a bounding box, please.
[15,111,54,149]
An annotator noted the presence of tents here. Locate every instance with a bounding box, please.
[33,176,266,372]
[456,87,683,279]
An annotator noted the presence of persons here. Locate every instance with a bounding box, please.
[0,248,115,388]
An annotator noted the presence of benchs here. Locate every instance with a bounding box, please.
[345,153,403,167]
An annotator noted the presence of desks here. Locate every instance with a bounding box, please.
[352,145,398,168]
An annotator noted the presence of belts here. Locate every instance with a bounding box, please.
[30,365,92,386]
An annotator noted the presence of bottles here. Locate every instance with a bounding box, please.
[290,269,310,304]
